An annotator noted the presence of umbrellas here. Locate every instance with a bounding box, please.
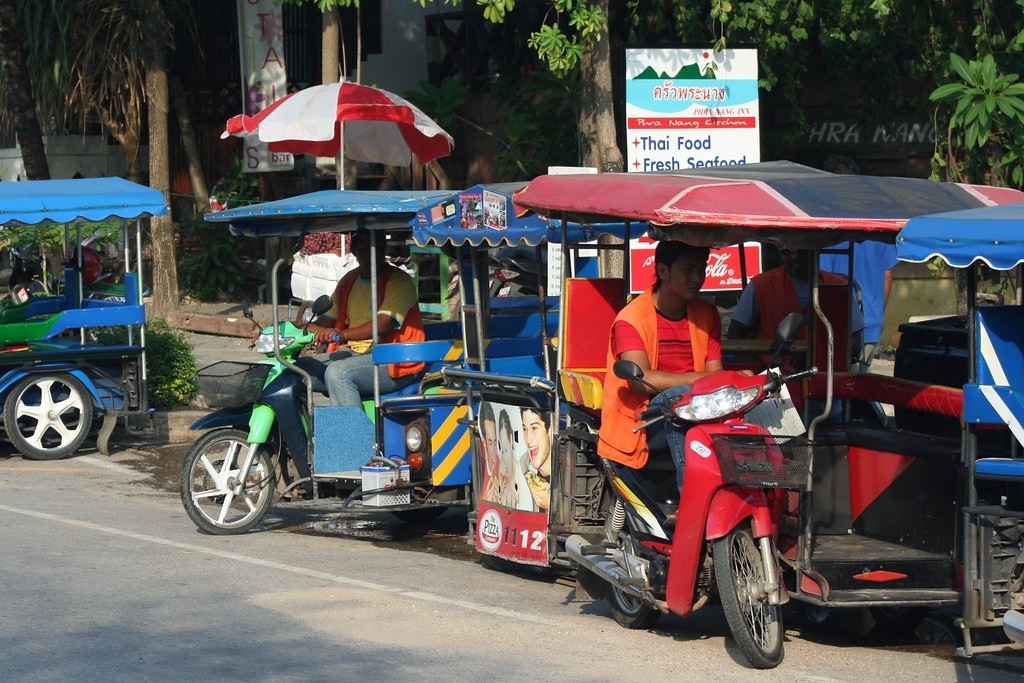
[221,82,453,254]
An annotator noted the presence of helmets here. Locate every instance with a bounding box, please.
[81,246,102,286]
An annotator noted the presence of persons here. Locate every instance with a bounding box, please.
[477,401,551,513]
[728,245,867,421]
[251,231,426,411]
[597,240,755,502]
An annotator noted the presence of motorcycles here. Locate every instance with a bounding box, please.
[177,161,1024,681]
[0,175,173,461]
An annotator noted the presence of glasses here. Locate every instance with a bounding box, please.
[782,249,808,256]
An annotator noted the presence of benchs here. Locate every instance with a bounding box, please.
[28,335,103,351]
[560,278,803,414]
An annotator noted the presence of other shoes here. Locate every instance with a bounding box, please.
[663,508,678,527]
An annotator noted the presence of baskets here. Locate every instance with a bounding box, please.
[196,361,275,411]
[711,433,818,489]
